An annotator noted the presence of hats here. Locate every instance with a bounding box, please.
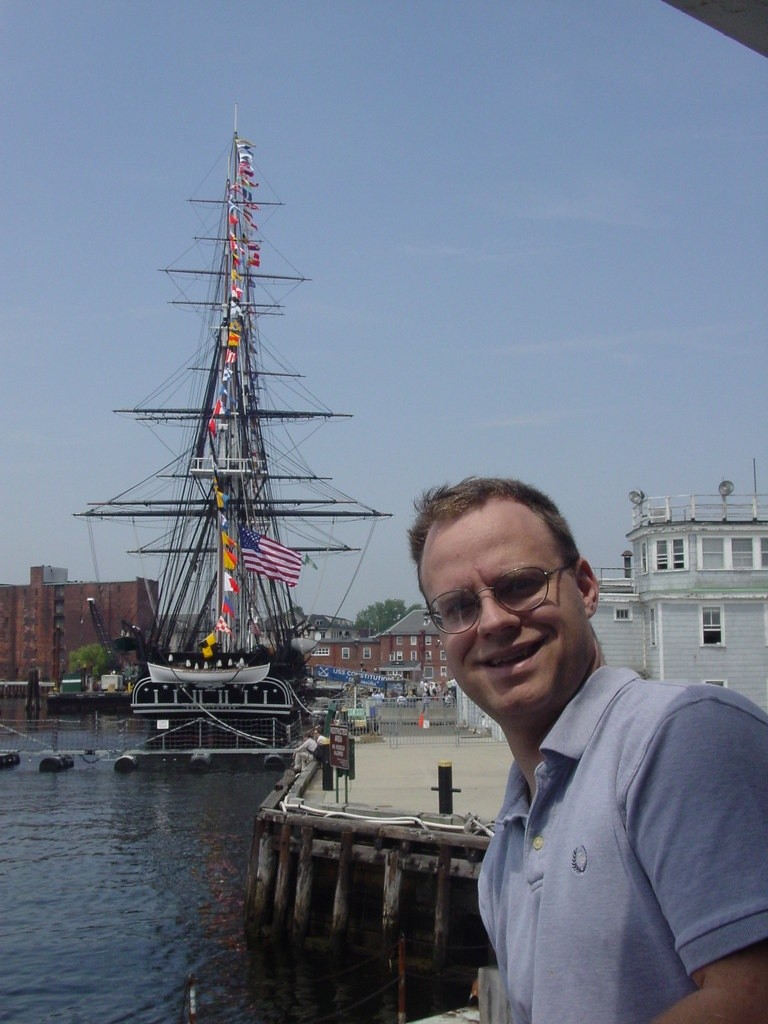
[303,732,311,738]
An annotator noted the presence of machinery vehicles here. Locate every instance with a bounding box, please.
[87,597,122,672]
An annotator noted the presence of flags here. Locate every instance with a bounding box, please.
[208,298,243,439]
[237,138,260,186]
[200,464,241,659]
[241,522,302,586]
[224,180,262,300]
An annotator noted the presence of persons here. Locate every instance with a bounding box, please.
[407,477,767,1024]
[313,729,330,747]
[372,687,417,708]
[292,732,323,772]
[420,677,456,714]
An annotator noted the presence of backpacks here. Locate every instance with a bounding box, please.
[313,746,322,760]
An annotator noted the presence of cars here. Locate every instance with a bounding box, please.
[346,709,379,733]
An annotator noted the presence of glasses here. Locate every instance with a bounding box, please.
[422,561,573,634]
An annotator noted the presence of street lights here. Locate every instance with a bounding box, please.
[345,682,357,716]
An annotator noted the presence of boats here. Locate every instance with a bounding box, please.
[83,100,400,744]
